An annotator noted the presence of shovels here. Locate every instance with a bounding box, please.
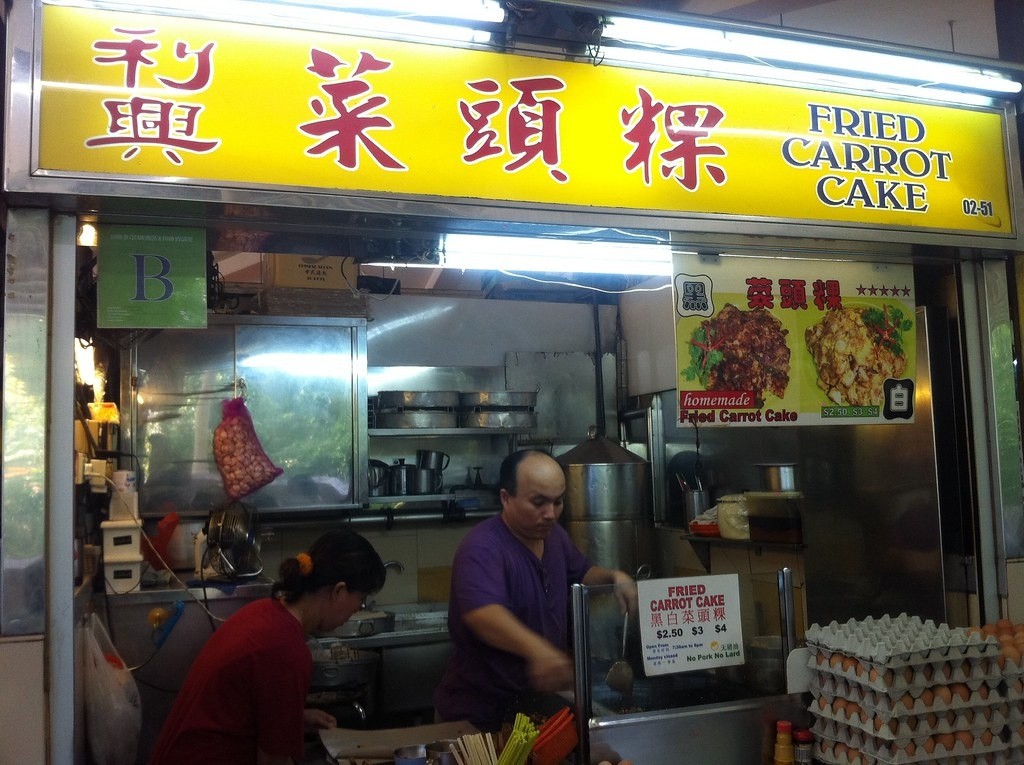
[603,610,635,697]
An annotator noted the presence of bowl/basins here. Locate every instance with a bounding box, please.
[689,523,719,537]
[530,707,578,765]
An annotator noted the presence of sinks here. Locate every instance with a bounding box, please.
[393,612,448,630]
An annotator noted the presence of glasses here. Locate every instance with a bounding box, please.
[347,583,366,614]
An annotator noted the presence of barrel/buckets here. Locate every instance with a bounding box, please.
[760,465,804,492]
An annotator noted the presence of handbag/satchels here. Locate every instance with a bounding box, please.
[82,613,142,765]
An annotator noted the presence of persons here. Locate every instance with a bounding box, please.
[431,447,638,724]
[147,527,386,765]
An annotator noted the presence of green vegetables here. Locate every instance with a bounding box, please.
[860,304,914,357]
[680,326,723,387]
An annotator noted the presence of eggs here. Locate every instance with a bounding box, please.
[816,619,1024,765]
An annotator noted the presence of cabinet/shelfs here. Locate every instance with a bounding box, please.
[365,426,542,512]
[119,315,368,517]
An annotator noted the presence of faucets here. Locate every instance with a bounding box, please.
[361,560,404,612]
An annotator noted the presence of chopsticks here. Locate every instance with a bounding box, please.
[450,713,539,765]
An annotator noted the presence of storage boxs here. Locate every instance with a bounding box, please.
[209,251,359,293]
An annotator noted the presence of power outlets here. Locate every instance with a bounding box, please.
[76,453,92,484]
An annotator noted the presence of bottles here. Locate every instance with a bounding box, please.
[774,721,793,765]
[794,729,813,765]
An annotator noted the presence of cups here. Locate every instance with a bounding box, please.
[388,457,415,495]
[368,460,389,496]
[717,495,751,539]
[425,739,465,765]
[683,489,709,531]
[415,450,450,471]
[393,744,427,765]
[414,468,444,494]
